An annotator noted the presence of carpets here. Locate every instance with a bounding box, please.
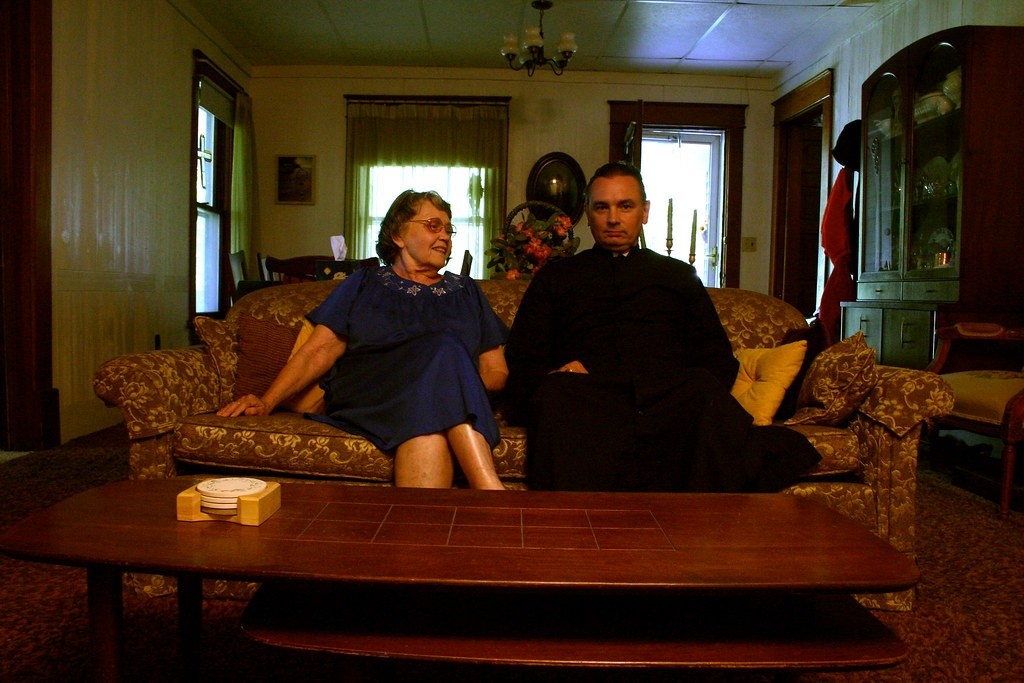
[0,425,1024,683]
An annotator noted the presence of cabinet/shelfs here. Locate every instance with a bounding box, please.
[835,21,1024,374]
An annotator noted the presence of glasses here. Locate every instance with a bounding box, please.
[407,218,457,237]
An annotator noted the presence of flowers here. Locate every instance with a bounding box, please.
[484,211,581,277]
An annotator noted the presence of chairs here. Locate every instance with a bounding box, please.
[227,247,250,299]
[911,310,1024,519]
[237,279,283,299]
[265,255,335,283]
[459,250,474,277]
[256,252,282,281]
[314,256,381,280]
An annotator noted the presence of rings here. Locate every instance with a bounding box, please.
[569,368,577,373]
[232,401,241,405]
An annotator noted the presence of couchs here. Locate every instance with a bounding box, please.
[92,263,956,617]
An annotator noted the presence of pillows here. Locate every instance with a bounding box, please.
[788,328,877,425]
[735,333,812,425]
[235,307,295,404]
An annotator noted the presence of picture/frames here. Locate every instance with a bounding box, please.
[272,153,318,206]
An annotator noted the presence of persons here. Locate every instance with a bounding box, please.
[216,190,510,490]
[499,161,821,494]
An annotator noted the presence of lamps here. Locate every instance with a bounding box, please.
[499,0,582,74]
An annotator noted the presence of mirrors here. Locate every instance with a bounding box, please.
[525,151,588,228]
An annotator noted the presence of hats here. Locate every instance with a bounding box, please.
[832,120,861,172]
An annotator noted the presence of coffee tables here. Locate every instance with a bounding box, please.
[0,471,928,683]
